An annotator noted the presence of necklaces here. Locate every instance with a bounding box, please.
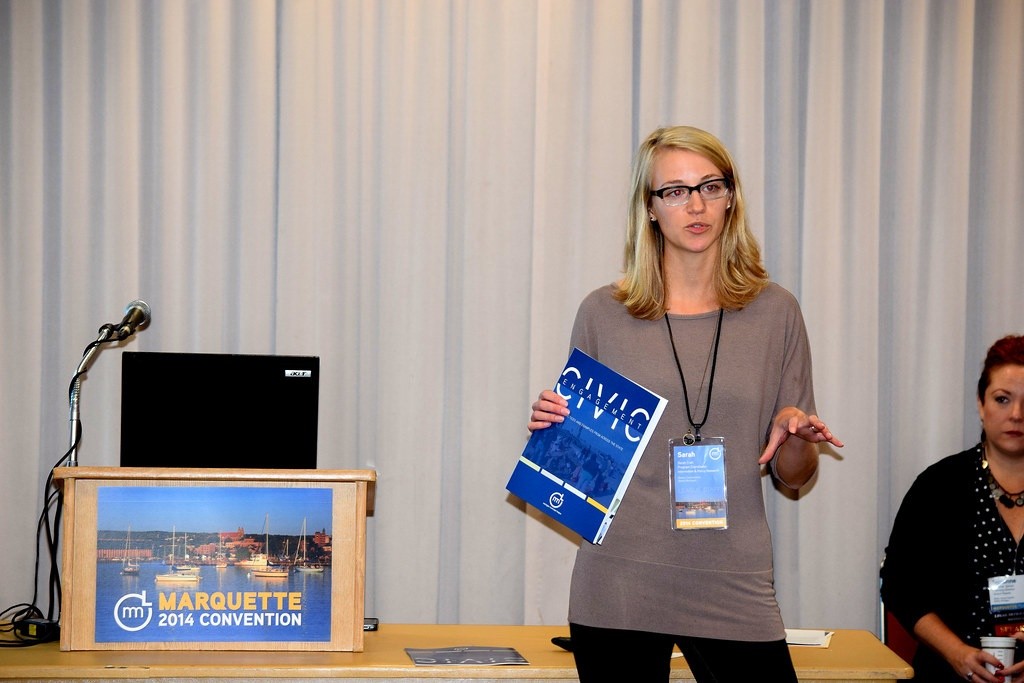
[981,443,1024,510]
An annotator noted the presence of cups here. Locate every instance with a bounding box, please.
[980,637,1016,683]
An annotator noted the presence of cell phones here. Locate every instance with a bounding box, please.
[363,618,377,631]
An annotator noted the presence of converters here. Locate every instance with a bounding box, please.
[20,618,60,639]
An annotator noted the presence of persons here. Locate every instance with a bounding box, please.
[879,336,1024,683]
[528,124,847,683]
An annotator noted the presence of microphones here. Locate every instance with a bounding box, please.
[117,301,151,340]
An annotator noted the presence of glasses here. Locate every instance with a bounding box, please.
[649,177,729,207]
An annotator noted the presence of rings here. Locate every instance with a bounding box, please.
[967,672,975,680]
[811,426,818,432]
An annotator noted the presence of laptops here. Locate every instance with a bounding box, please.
[121,351,320,470]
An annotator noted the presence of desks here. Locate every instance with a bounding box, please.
[0,624,914,683]
